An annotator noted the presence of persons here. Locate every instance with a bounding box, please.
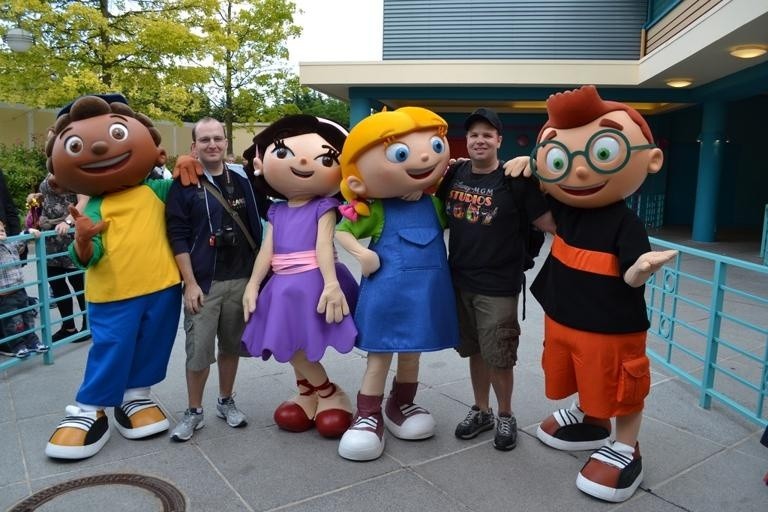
[0,167,30,355]
[225,153,236,165]
[44,90,204,462]
[241,113,361,438]
[240,154,248,166]
[503,82,680,502]
[164,114,263,441]
[335,105,469,463]
[0,221,50,359]
[25,171,92,342]
[147,147,172,180]
[437,107,559,451]
[755,423,768,485]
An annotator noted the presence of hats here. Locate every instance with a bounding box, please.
[465,108,503,132]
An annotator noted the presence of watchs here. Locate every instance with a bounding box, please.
[64,218,75,227]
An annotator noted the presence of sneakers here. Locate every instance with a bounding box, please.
[171,413,205,441]
[493,415,517,449]
[216,401,248,427]
[456,408,494,438]
[0,326,91,357]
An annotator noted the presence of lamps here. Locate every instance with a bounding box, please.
[728,45,767,59]
[665,79,692,89]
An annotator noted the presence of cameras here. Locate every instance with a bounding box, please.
[209,226,240,248]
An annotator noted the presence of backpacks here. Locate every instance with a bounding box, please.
[438,163,545,269]
